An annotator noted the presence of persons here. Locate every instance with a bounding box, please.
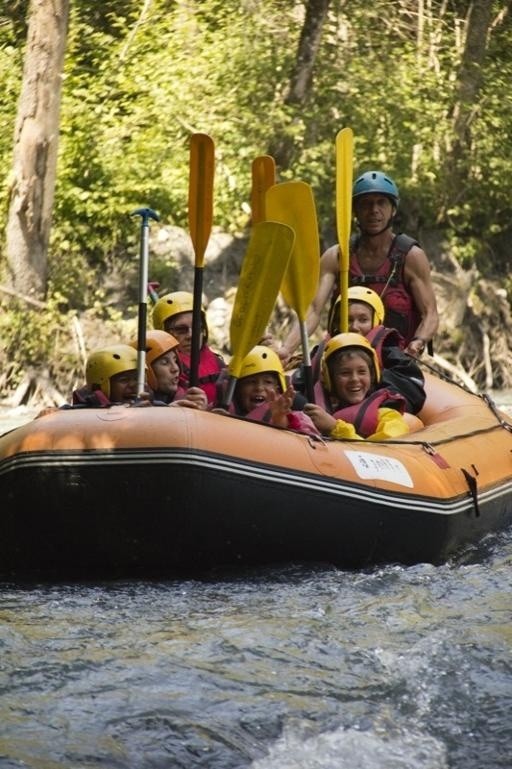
[227,344,321,438]
[303,332,410,441]
[275,171,440,369]
[33,344,155,420]
[150,291,273,413]
[128,329,210,409]
[290,285,427,416]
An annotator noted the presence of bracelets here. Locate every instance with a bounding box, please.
[411,337,426,343]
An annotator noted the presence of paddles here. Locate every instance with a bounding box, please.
[221,222,295,411]
[189,134,215,396]
[252,155,276,337]
[263,182,320,403]
[336,128,354,333]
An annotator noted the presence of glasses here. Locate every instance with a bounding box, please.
[113,374,148,383]
[166,324,204,335]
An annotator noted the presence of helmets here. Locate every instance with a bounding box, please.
[228,344,287,395]
[151,290,209,342]
[129,331,183,391]
[353,170,400,214]
[84,345,148,399]
[320,333,382,392]
[329,285,384,333]
